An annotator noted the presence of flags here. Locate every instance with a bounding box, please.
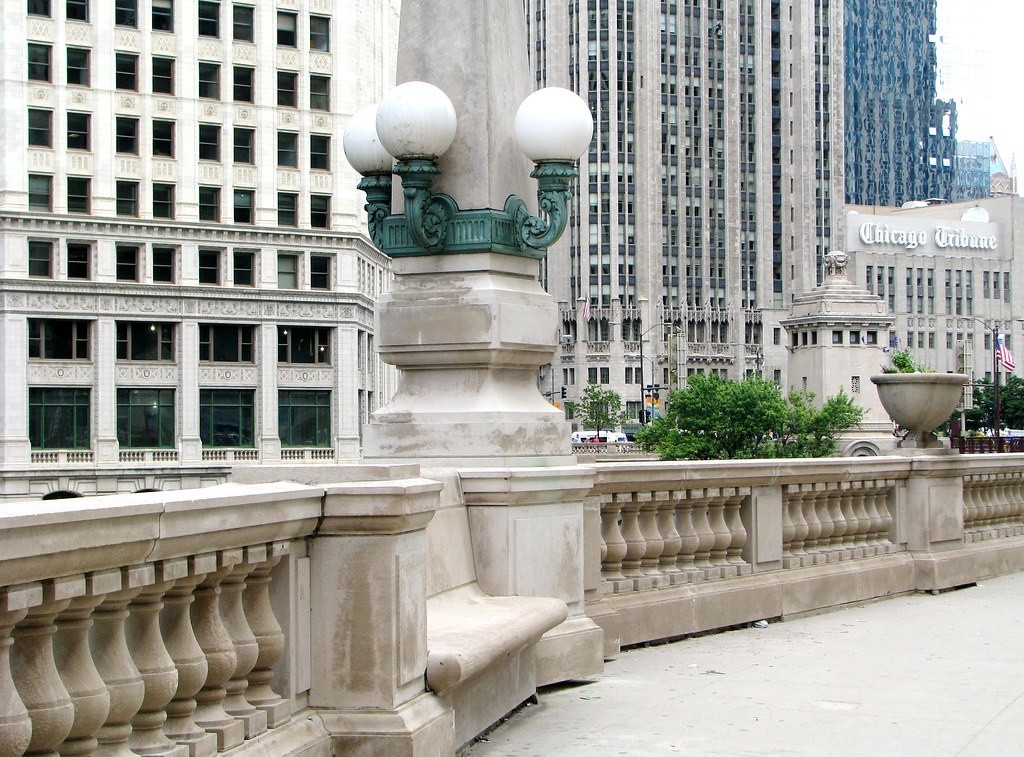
[996,338,1016,372]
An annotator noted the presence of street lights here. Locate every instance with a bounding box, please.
[636,354,655,427]
[962,316,1024,436]
[607,319,674,425]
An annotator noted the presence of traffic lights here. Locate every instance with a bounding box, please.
[638,409,644,423]
[560,386,568,399]
[645,410,651,423]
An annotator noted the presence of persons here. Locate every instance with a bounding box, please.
[949,417,961,448]
[573,433,599,449]
[1005,430,1013,444]
